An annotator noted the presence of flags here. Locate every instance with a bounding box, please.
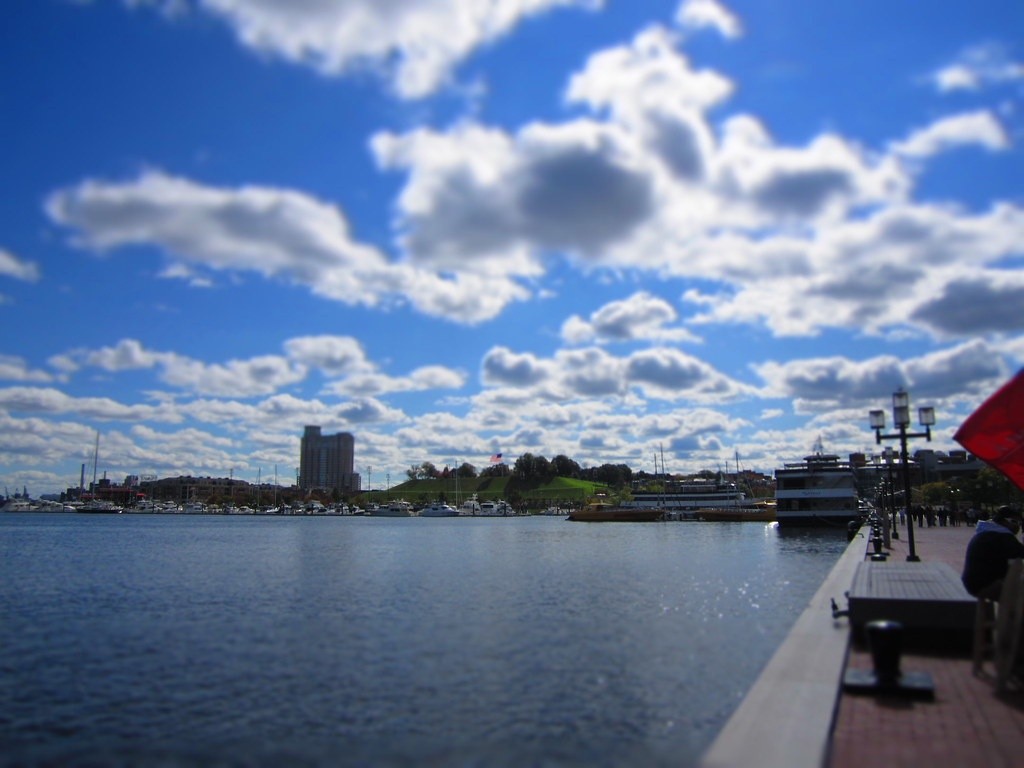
[490,454,502,463]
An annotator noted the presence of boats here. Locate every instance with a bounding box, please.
[619,473,777,520]
[76,500,163,514]
[375,498,516,517]
[774,452,863,527]
[565,441,776,522]
[858,500,869,513]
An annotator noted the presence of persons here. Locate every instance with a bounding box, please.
[900,506,906,525]
[937,507,961,527]
[966,506,999,526]
[911,505,937,527]
[961,505,1024,676]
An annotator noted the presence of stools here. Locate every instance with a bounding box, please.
[972,590,997,678]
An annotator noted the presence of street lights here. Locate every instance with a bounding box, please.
[871,447,908,539]
[869,386,935,562]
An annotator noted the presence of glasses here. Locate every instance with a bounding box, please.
[1004,518,1022,526]
